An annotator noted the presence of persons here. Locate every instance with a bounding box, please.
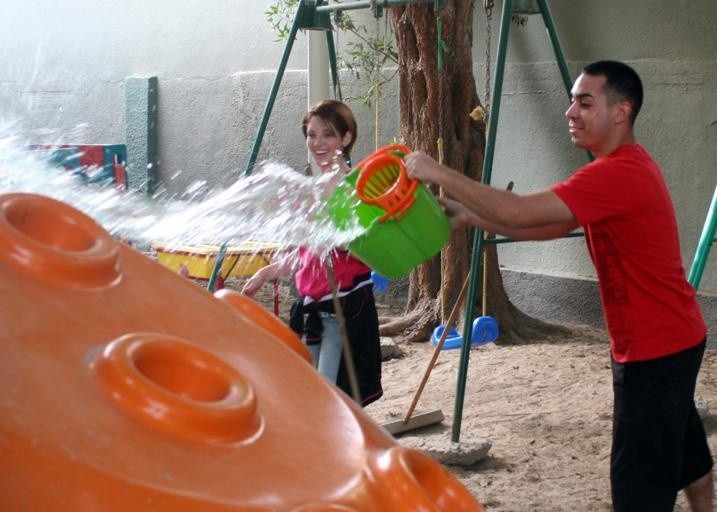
[239,98,384,409]
[401,57,715,512]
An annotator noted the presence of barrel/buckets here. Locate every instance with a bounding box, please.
[318,144,449,280]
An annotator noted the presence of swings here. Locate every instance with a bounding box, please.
[368,3,498,351]
